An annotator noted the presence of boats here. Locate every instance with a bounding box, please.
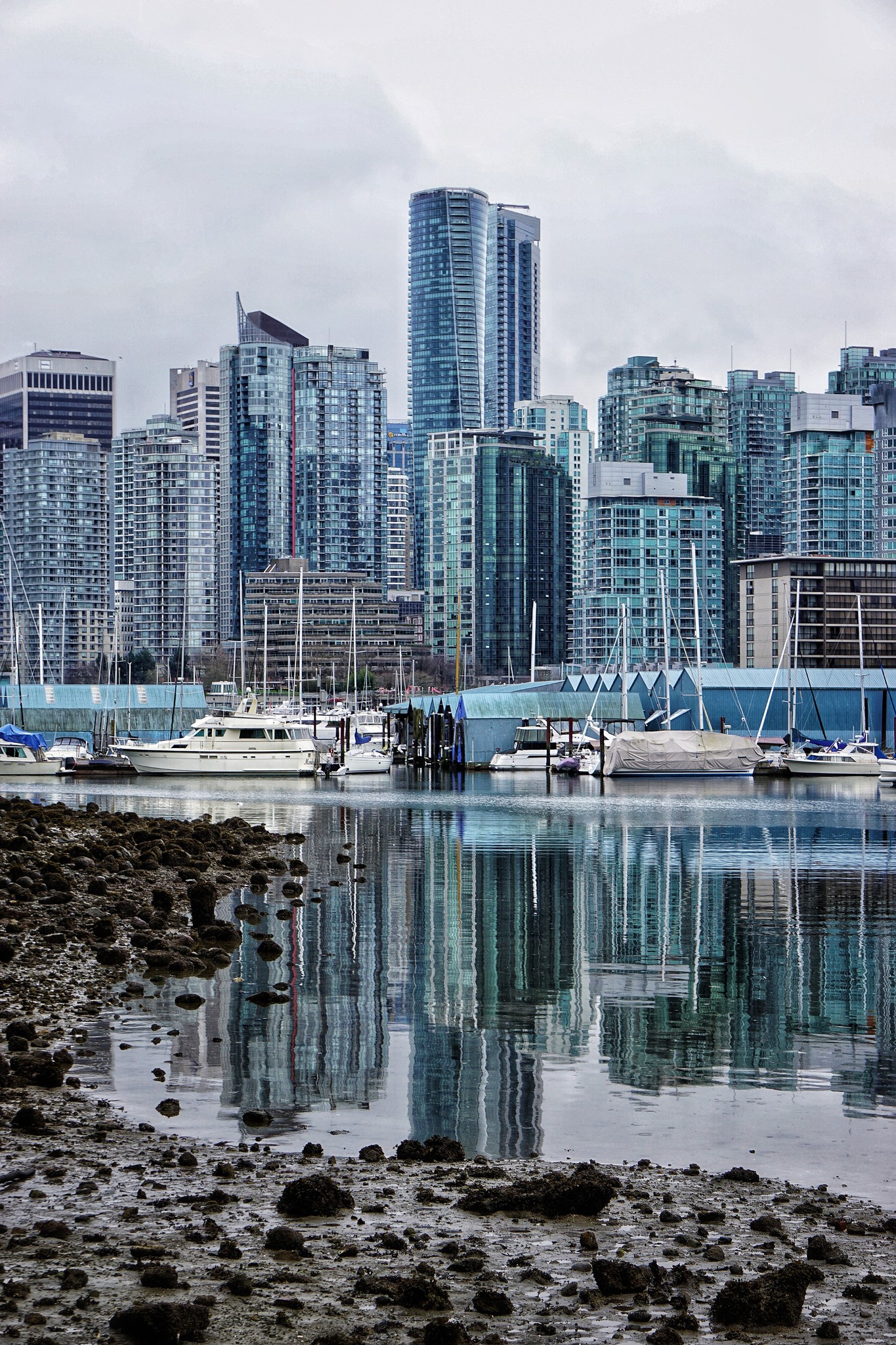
[0,519,896,778]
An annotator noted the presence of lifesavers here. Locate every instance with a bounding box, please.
[330,748,333,757]
